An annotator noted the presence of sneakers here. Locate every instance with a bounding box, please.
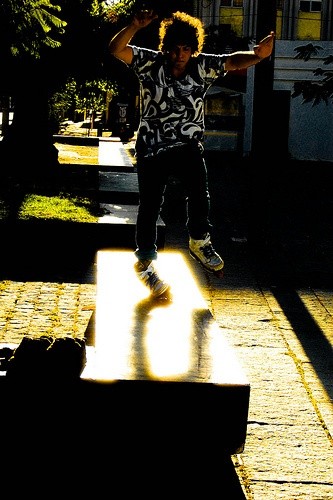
[188,234,224,273]
[134,260,169,297]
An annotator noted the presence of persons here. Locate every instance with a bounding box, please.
[108,7,276,298]
